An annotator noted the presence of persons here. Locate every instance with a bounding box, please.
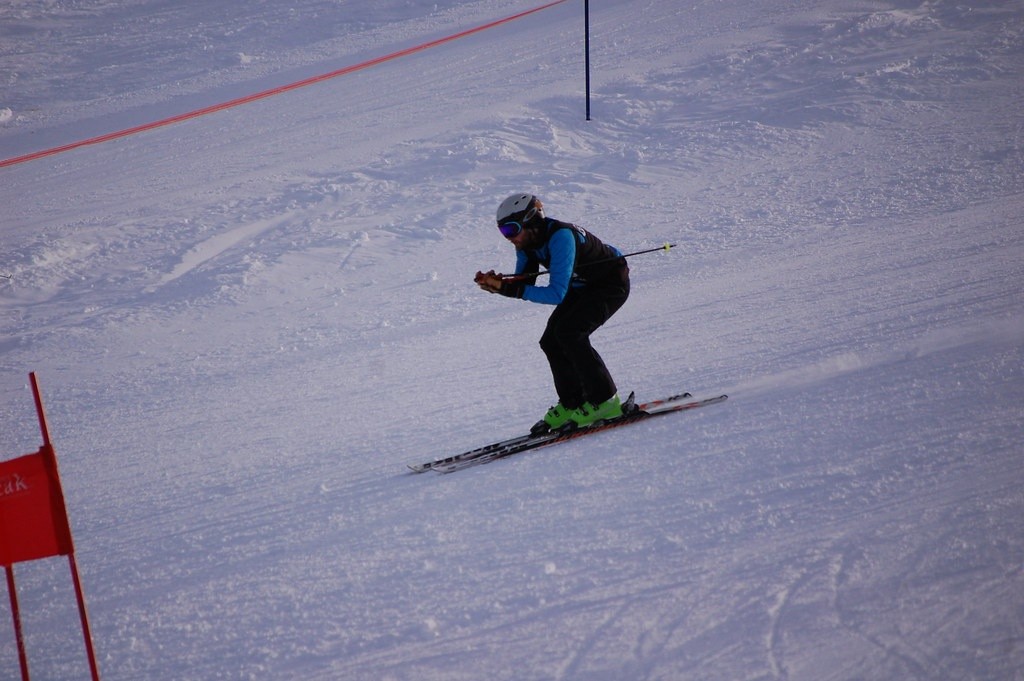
[473,194,631,432]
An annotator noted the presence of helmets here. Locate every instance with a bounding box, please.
[497,192,545,228]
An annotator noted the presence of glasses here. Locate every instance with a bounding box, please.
[497,220,522,239]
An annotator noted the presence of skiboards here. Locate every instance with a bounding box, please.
[405,388,727,475]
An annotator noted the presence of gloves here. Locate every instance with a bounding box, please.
[476,269,525,299]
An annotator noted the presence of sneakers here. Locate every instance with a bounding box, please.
[545,393,623,429]
